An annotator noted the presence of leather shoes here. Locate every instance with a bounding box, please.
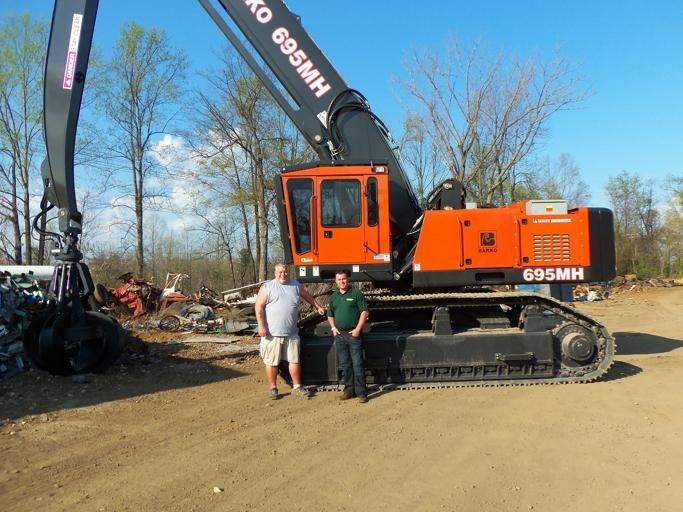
[358,395,369,403]
[338,392,355,400]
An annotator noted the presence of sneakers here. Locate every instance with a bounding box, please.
[290,385,314,398]
[269,387,279,400]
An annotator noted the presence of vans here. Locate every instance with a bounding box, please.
[0,264,55,291]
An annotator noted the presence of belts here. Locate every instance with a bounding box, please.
[337,329,356,335]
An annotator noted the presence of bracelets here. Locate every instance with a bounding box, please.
[330,327,335,331]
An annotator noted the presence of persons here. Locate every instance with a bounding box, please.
[255,262,325,399]
[326,269,371,402]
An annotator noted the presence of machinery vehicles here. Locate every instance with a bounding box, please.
[25,0,619,393]
